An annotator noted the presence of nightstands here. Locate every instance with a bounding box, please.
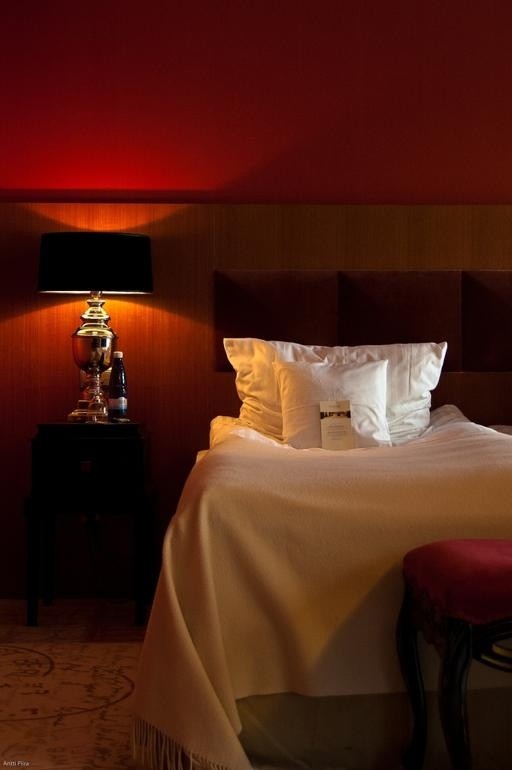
[22,423,158,627]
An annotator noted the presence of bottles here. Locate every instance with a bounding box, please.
[106,349,128,419]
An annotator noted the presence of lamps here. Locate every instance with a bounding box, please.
[34,227,156,425]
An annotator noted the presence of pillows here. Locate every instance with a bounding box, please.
[223,337,447,451]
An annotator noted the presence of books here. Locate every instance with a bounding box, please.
[319,399,354,450]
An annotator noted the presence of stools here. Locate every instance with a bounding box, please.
[391,535,510,769]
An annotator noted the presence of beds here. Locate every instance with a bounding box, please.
[196,268,512,770]
